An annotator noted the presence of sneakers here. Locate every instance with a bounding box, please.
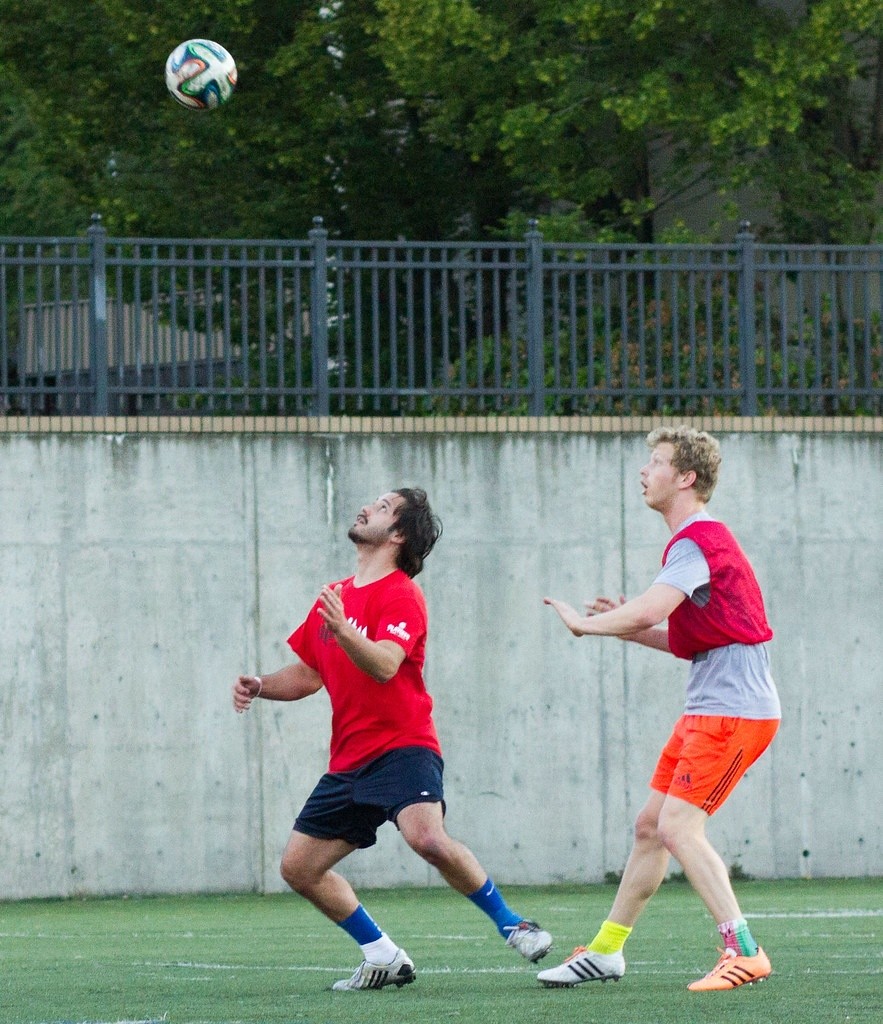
[331,949,416,992]
[688,947,771,990]
[537,946,625,988]
[505,920,554,965]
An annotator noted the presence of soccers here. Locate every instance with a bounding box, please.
[163,37,239,114]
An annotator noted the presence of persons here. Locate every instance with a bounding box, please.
[536,426,782,990]
[233,488,554,989]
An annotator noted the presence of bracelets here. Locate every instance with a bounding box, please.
[255,677,262,697]
